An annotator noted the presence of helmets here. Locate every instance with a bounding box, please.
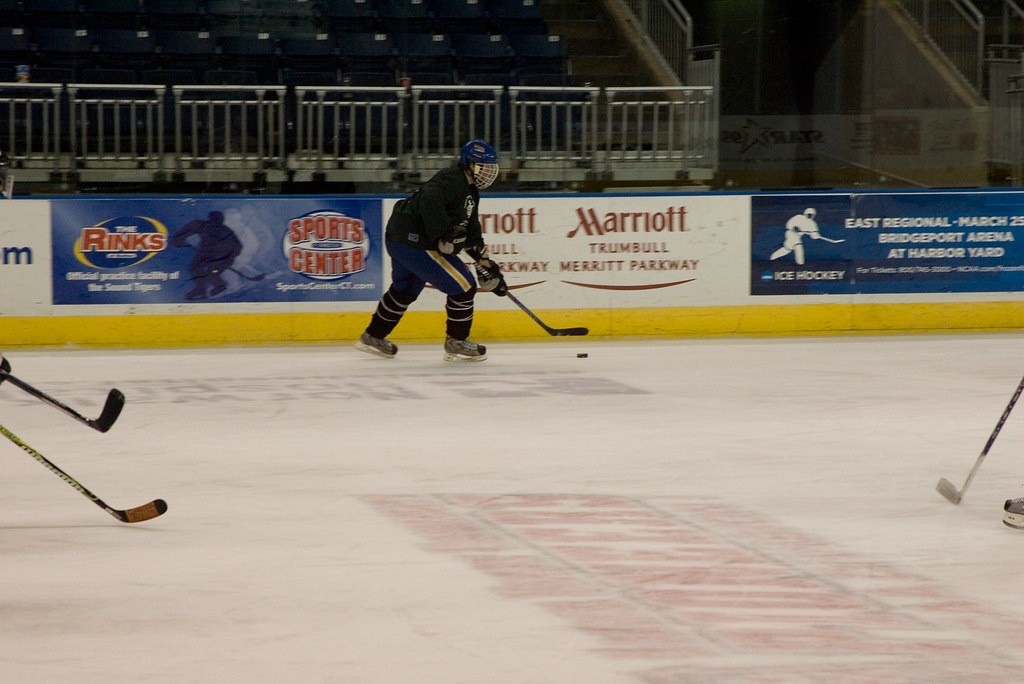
[459,139,499,189]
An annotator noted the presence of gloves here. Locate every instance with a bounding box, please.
[478,260,502,291]
[437,226,466,256]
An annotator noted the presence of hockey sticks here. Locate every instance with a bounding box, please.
[0,423,169,524]
[0,350,127,435]
[443,237,589,338]
[936,379,1024,505]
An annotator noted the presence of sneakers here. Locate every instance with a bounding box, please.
[443,335,487,363]
[1003,498,1024,529]
[354,330,398,358]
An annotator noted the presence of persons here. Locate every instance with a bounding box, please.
[354,139,507,363]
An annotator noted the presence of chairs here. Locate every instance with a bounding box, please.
[0,0,593,151]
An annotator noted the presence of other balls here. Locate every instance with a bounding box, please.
[576,352,589,359]
